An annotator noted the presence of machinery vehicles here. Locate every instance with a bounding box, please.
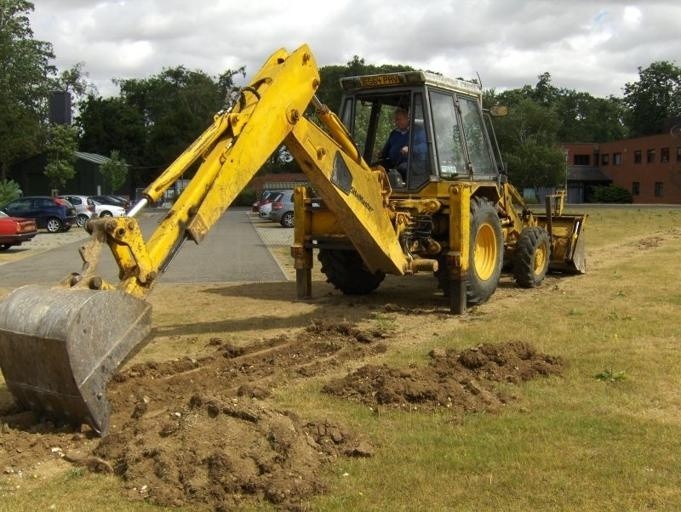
[0,41,591,435]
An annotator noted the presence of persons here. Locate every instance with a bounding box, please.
[370,108,427,181]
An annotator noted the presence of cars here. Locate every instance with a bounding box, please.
[0,208,39,254]
[2,192,136,234]
[248,184,320,229]
